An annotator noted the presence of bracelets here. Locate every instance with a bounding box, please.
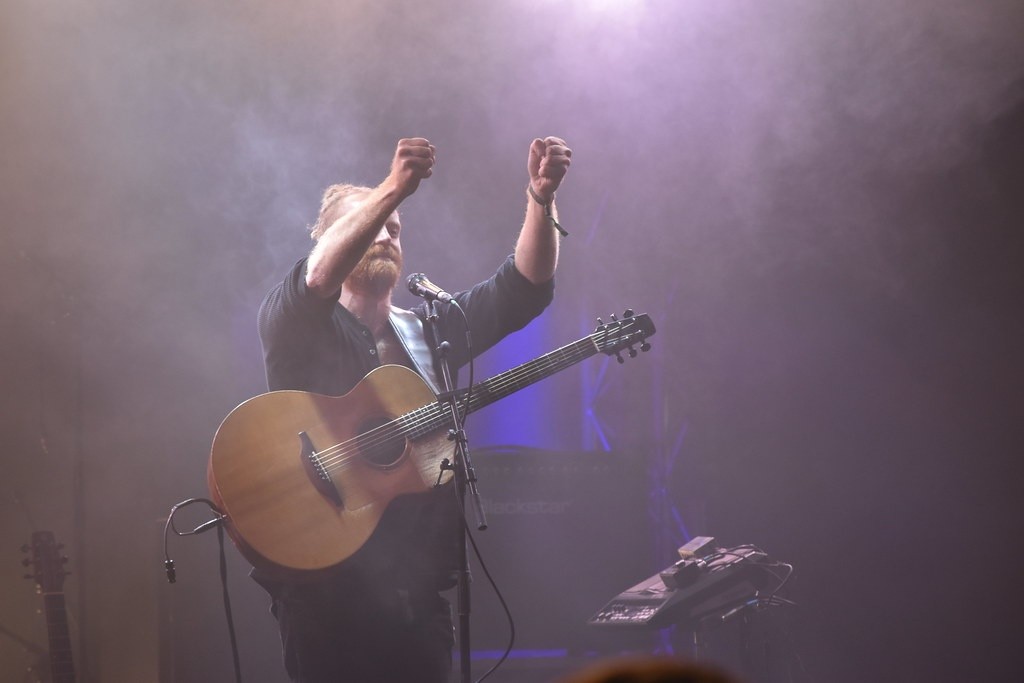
[529,183,568,238]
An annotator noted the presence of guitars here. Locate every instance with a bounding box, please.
[19,531,84,683]
[205,304,661,592]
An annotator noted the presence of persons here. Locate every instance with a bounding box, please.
[258,136,572,683]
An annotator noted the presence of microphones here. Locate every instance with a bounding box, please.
[406,273,454,303]
